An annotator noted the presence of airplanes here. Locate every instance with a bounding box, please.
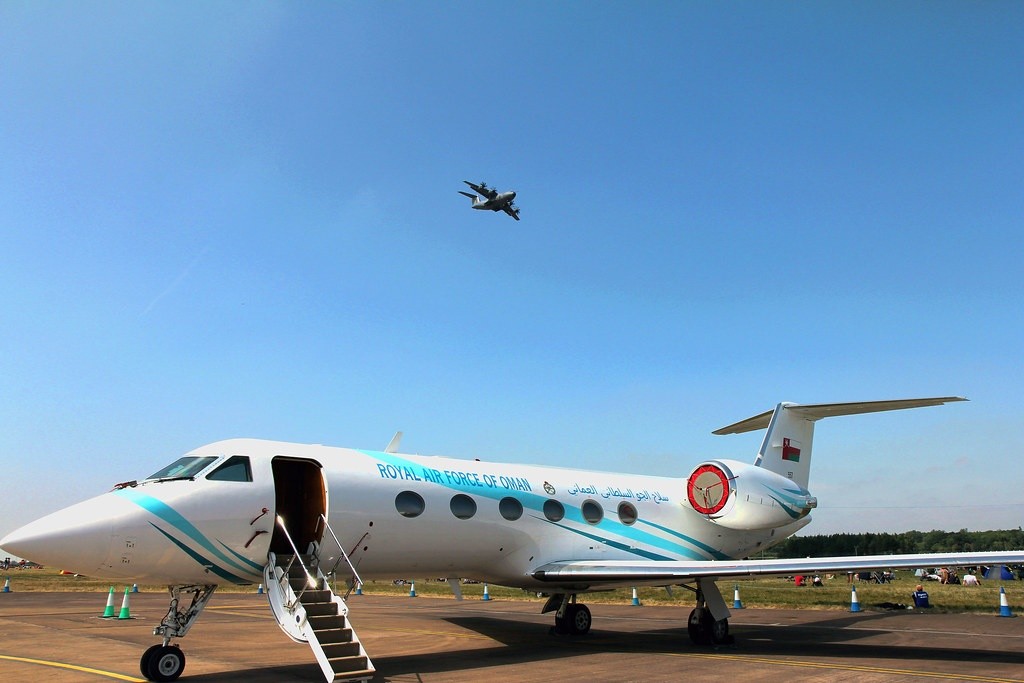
[0,396,1024,683]
[458,180,521,221]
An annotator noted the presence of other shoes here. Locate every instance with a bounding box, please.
[811,584,814,586]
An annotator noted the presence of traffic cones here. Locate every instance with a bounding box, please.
[629,587,643,606]
[480,583,493,601]
[113,586,136,620]
[98,586,119,618]
[995,587,1018,618]
[130,582,141,593]
[729,584,746,609]
[354,582,364,595]
[255,583,266,594]
[1,575,13,593]
[846,585,865,613]
[409,579,418,597]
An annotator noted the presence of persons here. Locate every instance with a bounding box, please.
[2,556,12,570]
[786,574,833,587]
[846,569,896,586]
[911,584,935,608]
[936,567,982,587]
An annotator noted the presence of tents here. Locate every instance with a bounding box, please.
[985,566,1015,581]
